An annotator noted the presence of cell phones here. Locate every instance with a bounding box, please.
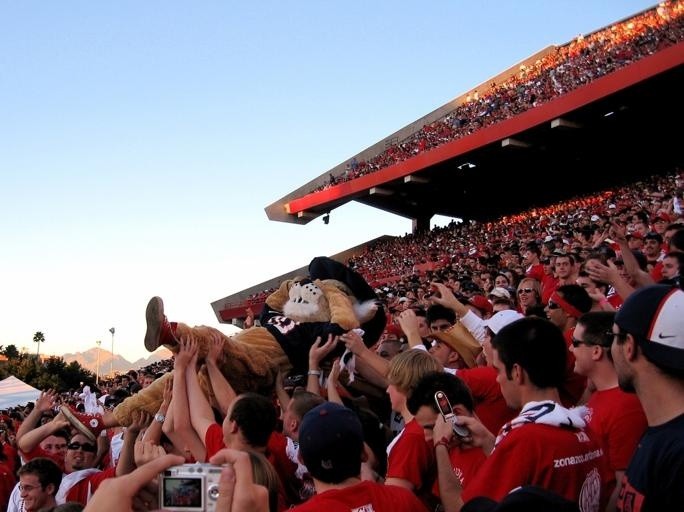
[435,391,470,439]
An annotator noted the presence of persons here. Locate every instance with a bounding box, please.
[314,1,684,288]
[242,289,684,512]
[58,257,387,444]
[0,330,242,512]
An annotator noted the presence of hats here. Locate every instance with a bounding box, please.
[614,283,684,350]
[481,310,526,334]
[489,288,510,298]
[299,402,362,470]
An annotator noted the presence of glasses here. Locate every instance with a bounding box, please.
[519,288,535,293]
[548,301,560,308]
[572,337,598,347]
[68,442,94,451]
[606,329,623,345]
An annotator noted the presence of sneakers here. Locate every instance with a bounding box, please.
[145,296,177,351]
[61,406,105,441]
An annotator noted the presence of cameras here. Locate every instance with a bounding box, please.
[158,464,223,512]
